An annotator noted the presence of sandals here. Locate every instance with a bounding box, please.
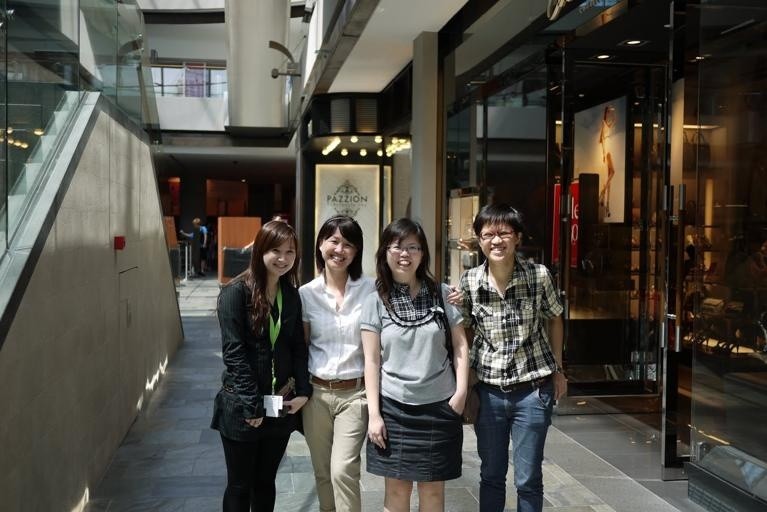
[606,207,610,217]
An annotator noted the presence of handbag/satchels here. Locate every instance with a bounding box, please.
[684,225,712,249]
[703,298,724,312]
[724,249,767,288]
[462,387,480,424]
[657,131,710,168]
[724,301,751,319]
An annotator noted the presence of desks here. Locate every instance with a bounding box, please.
[178,238,193,284]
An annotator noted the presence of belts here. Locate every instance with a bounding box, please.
[312,375,364,391]
[499,377,546,394]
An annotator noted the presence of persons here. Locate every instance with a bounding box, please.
[598,103,620,221]
[179,217,209,276]
[459,204,570,511]
[295,214,464,511]
[357,216,472,512]
[211,220,314,511]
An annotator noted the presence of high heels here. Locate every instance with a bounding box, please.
[599,191,605,207]
[686,328,709,345]
[711,336,739,353]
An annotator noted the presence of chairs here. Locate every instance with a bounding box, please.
[685,233,766,368]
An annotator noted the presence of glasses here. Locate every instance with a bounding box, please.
[479,229,515,239]
[387,245,423,253]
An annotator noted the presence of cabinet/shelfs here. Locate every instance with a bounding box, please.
[626,157,723,296]
